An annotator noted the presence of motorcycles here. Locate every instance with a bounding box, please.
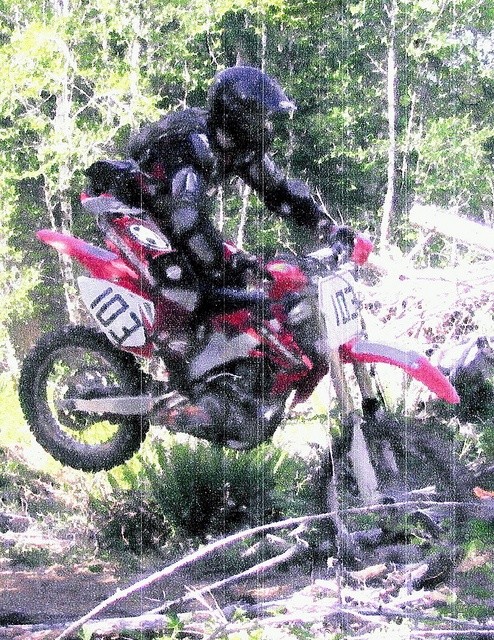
[18,160,477,593]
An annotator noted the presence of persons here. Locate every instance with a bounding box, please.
[78,63,354,381]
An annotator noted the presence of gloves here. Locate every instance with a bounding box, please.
[227,253,259,291]
[324,226,355,257]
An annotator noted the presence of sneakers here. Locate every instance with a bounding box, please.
[148,392,212,430]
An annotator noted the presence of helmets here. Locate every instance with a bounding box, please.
[207,67,295,162]
